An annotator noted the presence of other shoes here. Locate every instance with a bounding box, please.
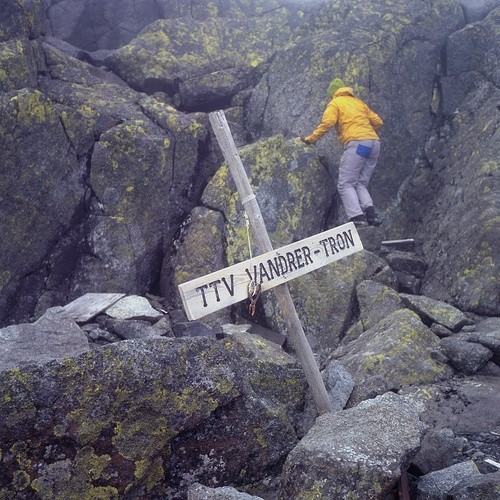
[365,207,381,221]
[350,216,365,224]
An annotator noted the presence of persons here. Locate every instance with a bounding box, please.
[300,78,384,229]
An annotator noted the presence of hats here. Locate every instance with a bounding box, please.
[327,79,344,97]
